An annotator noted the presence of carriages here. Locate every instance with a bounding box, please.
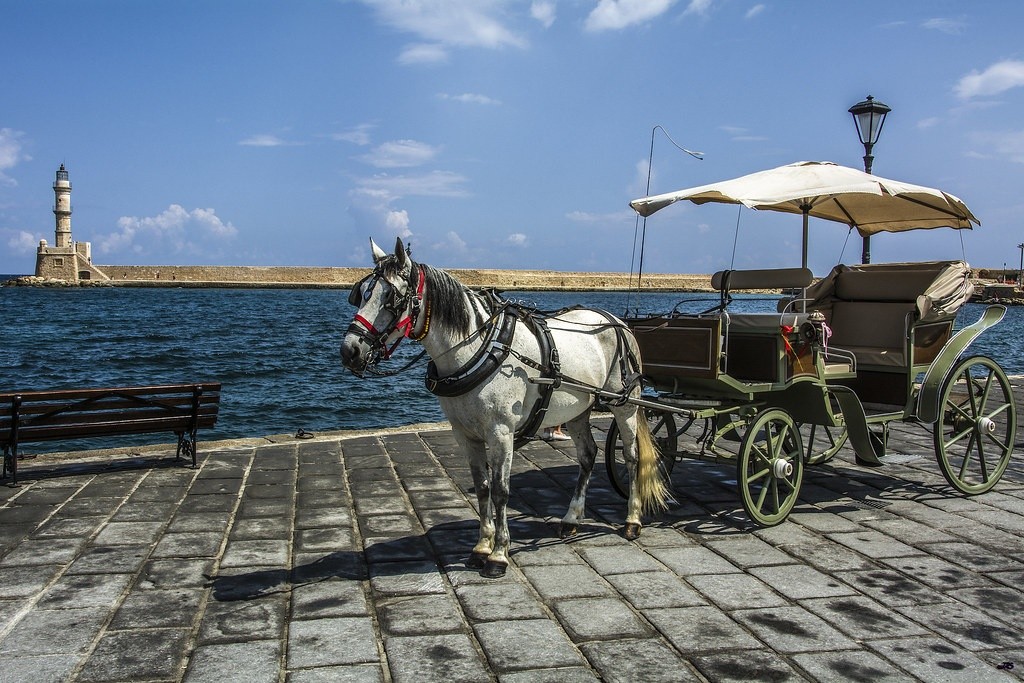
[340,158,1016,580]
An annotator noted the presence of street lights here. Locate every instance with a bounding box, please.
[846,94,893,264]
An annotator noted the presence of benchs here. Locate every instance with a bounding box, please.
[794,260,972,365]
[710,268,857,378]
[0,382,221,487]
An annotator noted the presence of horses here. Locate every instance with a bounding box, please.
[340,236,681,578]
